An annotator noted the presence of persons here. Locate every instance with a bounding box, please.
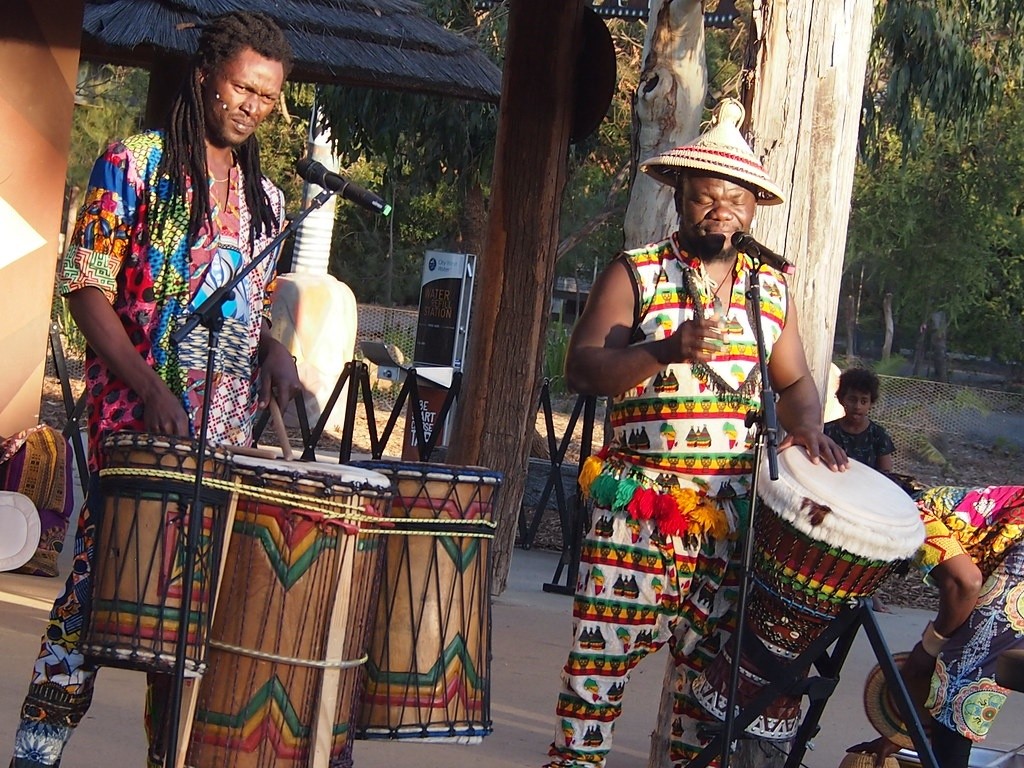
[550,99,851,768]
[817,365,894,489]
[881,480,1024,767]
[7,12,314,768]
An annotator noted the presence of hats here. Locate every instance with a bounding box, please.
[641,97,787,205]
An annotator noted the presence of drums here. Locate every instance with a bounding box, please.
[84,428,237,680]
[143,445,397,768]
[692,442,928,742]
[344,459,508,751]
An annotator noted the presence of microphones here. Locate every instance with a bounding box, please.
[731,231,795,275]
[297,158,392,216]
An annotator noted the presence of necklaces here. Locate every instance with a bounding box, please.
[707,259,738,318]
[210,176,228,184]
